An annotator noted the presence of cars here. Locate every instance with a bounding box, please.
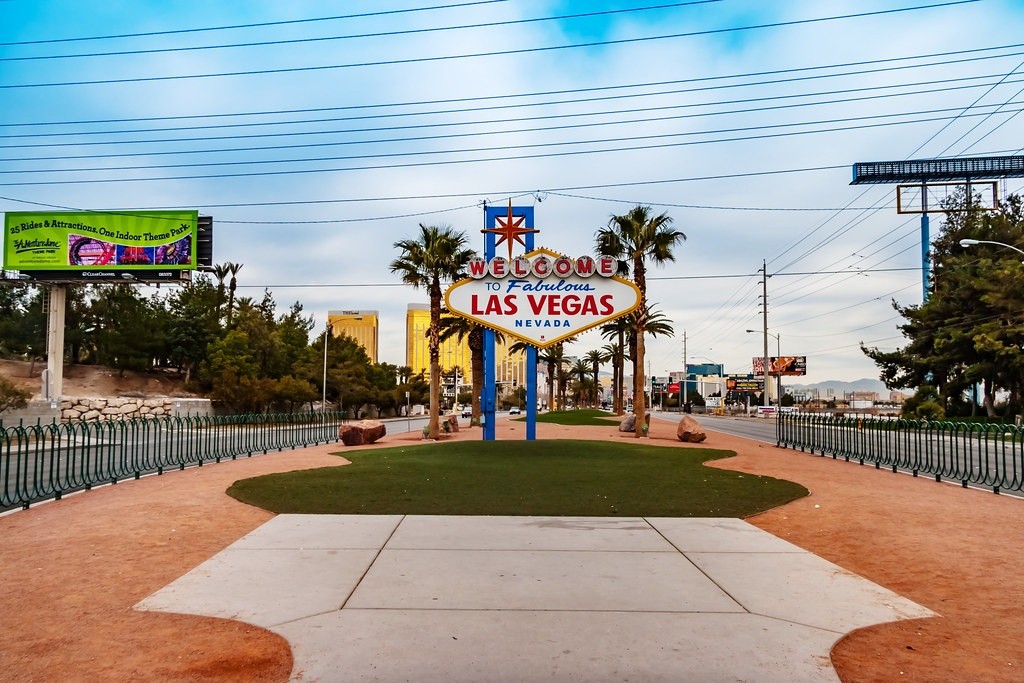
[598,404,634,413]
[509,406,520,414]
[561,401,577,411]
[461,407,473,418]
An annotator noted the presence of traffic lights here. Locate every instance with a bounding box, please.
[651,376,656,384]
[669,377,673,385]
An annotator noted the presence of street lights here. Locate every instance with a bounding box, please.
[319,317,364,442]
[690,356,723,408]
[746,329,781,415]
